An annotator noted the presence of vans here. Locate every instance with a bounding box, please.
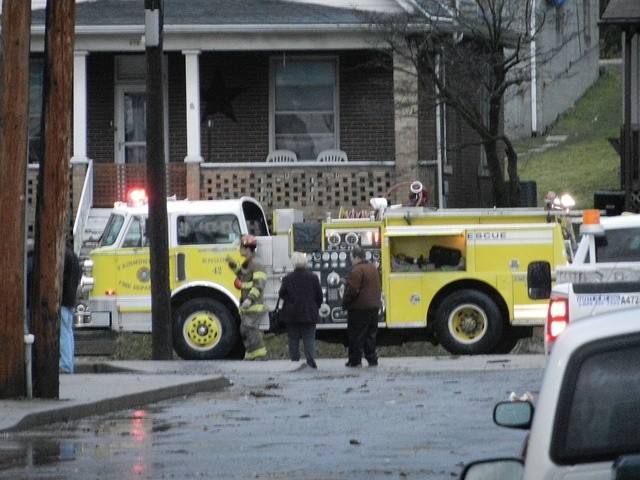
[493,308,640,480]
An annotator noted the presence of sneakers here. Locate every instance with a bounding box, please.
[308,361,317,368]
[345,361,362,368]
[368,362,378,367]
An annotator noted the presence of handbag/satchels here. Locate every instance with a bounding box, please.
[268,297,288,334]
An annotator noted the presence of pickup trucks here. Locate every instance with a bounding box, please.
[544,215,640,361]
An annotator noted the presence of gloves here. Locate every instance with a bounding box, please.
[242,298,252,308]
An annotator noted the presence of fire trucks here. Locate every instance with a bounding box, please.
[77,196,568,360]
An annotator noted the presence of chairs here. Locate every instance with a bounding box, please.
[260,149,297,201]
[310,149,348,202]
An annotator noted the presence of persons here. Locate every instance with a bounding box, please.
[59,233,83,374]
[278,251,324,370]
[341,247,382,367]
[226,234,268,361]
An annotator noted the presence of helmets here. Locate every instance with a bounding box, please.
[240,235,257,247]
